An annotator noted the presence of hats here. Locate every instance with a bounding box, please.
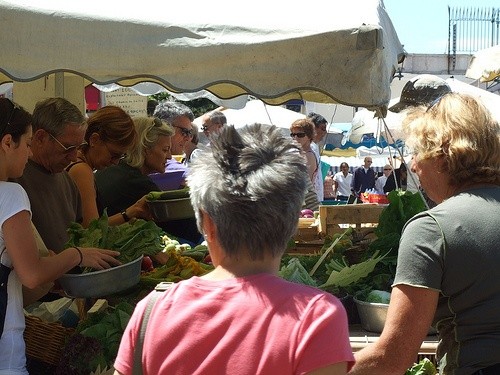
[388,74,453,113]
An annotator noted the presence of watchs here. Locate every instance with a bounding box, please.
[121,208,130,221]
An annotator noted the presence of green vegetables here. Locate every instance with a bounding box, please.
[279,190,431,306]
[64,207,165,274]
[403,357,437,375]
[60,294,141,375]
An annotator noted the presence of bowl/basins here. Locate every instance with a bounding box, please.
[145,197,194,222]
[353,289,439,337]
[57,253,145,300]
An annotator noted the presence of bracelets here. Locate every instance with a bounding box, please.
[73,246,83,266]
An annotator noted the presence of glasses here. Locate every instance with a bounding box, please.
[290,132,306,137]
[49,133,90,153]
[201,123,215,130]
[103,140,128,162]
[173,124,195,136]
[384,168,391,171]
[367,160,373,163]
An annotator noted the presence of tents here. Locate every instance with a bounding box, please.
[464,47,500,87]
[0,0,408,120]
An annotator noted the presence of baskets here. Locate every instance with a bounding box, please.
[24,284,86,364]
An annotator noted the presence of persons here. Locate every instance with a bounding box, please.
[181,125,200,168]
[387,75,453,194]
[348,92,500,375]
[202,110,226,139]
[154,101,195,155]
[64,106,156,230]
[290,111,327,204]
[112,124,356,375]
[91,114,174,222]
[334,155,423,206]
[0,98,119,375]
[7,97,89,254]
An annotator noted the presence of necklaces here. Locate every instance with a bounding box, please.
[189,147,196,160]
